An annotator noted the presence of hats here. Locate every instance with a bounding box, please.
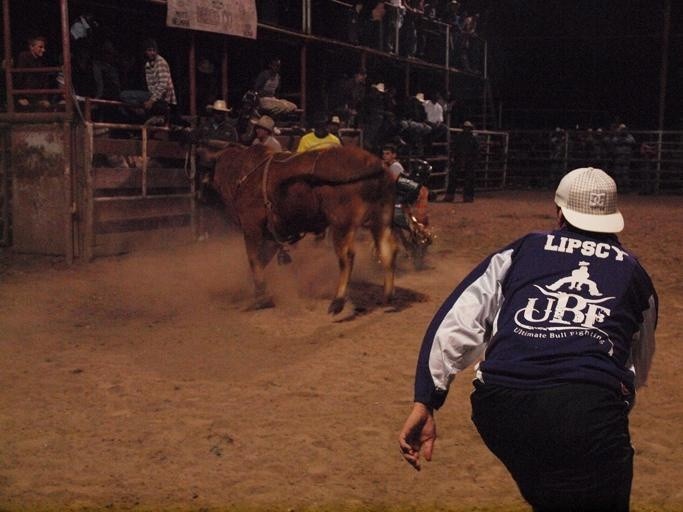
[330,116,340,123]
[250,114,281,135]
[371,82,384,93]
[463,121,472,128]
[143,38,158,50]
[206,100,232,113]
[412,93,425,102]
[555,124,626,133]
[554,167,625,234]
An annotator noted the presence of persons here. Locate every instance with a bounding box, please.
[205,100,344,153]
[443,121,480,202]
[252,59,297,98]
[352,0,478,56]
[508,124,635,192]
[14,38,51,108]
[120,39,177,122]
[344,72,444,271]
[398,167,658,512]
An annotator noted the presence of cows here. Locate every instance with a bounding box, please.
[193,143,397,316]
